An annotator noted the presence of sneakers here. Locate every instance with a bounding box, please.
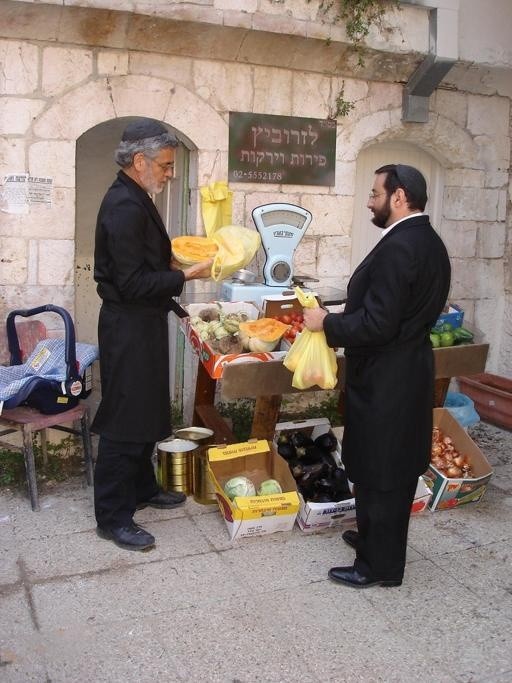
[97,524,154,550]
[136,489,186,509]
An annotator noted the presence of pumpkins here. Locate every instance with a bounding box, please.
[236,317,289,353]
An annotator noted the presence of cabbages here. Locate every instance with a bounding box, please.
[224,475,257,498]
[259,478,283,496]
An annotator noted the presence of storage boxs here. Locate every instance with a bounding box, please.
[205,406,495,541]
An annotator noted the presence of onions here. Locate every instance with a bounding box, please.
[431,426,476,480]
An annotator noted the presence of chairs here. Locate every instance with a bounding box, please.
[0,321,93,513]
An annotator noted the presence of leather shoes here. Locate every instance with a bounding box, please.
[329,530,402,588]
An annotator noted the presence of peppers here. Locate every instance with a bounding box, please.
[431,324,474,348]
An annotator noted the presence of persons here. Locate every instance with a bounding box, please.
[302,163,452,590]
[86,113,219,551]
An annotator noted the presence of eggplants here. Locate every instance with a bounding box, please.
[278,428,350,502]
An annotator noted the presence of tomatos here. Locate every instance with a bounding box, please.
[270,310,304,345]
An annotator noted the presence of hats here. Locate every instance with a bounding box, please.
[122,118,168,141]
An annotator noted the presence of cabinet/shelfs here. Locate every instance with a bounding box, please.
[183,301,492,444]
